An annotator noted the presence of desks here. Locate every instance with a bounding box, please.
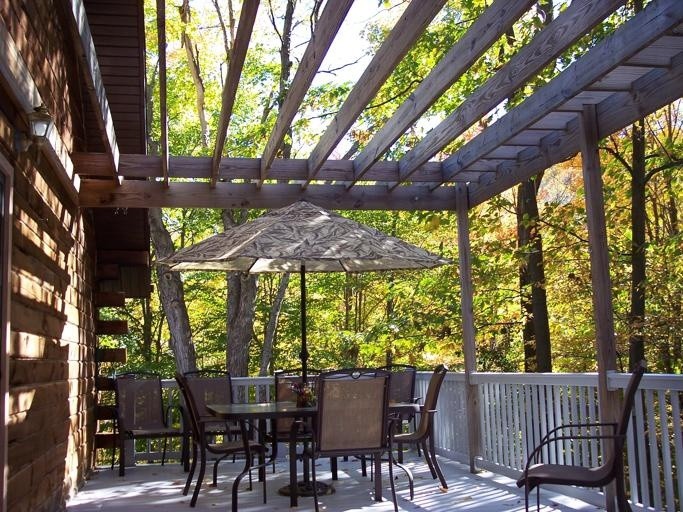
[203,399,425,511]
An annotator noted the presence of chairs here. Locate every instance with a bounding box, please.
[517,359,647,512]
[381,363,448,492]
[183,369,255,467]
[172,371,270,509]
[343,364,422,464]
[261,367,326,444]
[112,371,192,479]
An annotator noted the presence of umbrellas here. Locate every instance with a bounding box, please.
[155,198,456,482]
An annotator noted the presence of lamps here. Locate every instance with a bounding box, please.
[14,103,53,165]
